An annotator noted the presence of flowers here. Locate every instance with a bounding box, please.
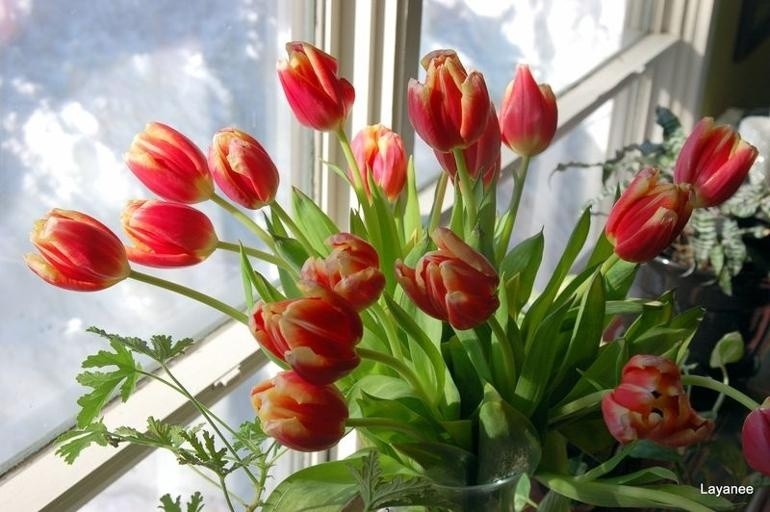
[25,41,770,511]
[547,105,769,297]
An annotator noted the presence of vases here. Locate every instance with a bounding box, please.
[602,255,687,353]
[410,424,532,511]
